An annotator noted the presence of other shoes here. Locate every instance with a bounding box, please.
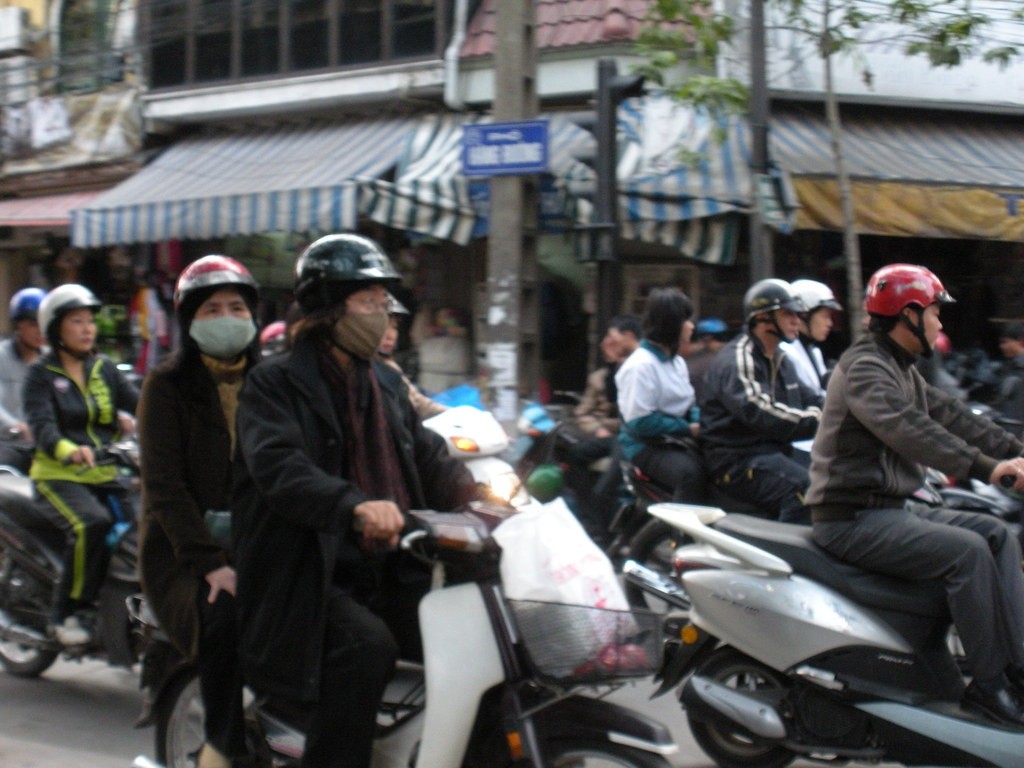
[54,617,90,645]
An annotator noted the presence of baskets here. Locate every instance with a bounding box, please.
[503,598,665,683]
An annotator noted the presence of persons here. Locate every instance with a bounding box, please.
[135,255,267,768]
[808,263,1024,727]
[0,288,47,476]
[684,316,728,390]
[616,286,704,583]
[989,323,1024,441]
[779,279,841,466]
[703,279,828,523]
[380,294,449,419]
[230,234,497,767]
[22,282,144,647]
[572,314,641,535]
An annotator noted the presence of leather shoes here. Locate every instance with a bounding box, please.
[960,679,1024,730]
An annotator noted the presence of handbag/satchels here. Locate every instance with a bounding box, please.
[489,494,639,677]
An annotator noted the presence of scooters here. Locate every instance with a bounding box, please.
[0,405,1024,767]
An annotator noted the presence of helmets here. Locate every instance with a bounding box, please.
[36,282,102,340]
[291,232,403,299]
[258,321,288,355]
[385,291,411,315]
[695,319,729,334]
[743,280,808,323]
[789,280,843,313]
[933,332,952,357]
[174,255,255,316]
[9,287,48,320]
[865,263,956,318]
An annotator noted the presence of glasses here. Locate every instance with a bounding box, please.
[345,295,393,315]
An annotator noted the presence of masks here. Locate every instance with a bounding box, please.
[319,309,388,359]
[189,315,257,359]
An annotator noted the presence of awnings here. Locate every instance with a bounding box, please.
[769,107,1024,241]
[0,188,105,226]
[72,109,439,249]
[365,96,755,263]
